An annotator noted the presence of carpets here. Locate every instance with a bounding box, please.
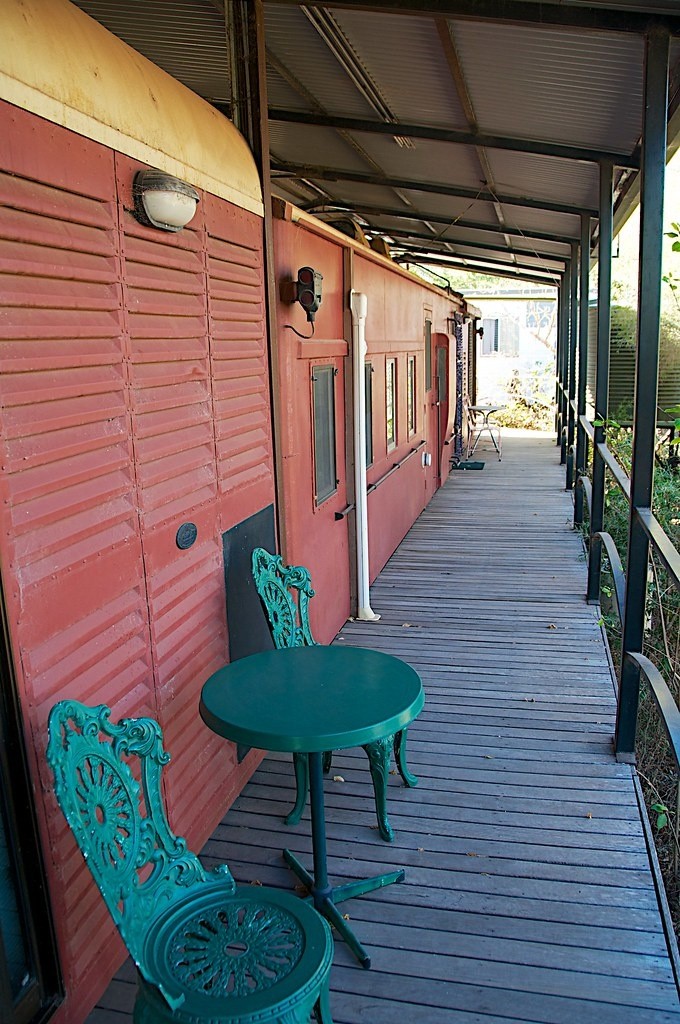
[458,462,485,470]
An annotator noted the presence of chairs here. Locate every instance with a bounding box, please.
[47,699,338,1022]
[247,548,421,843]
[462,395,502,452]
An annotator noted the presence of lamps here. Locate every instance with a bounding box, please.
[448,311,484,340]
[285,264,324,341]
[133,170,198,231]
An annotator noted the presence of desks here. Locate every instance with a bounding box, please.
[199,645,426,962]
[470,404,508,455]
